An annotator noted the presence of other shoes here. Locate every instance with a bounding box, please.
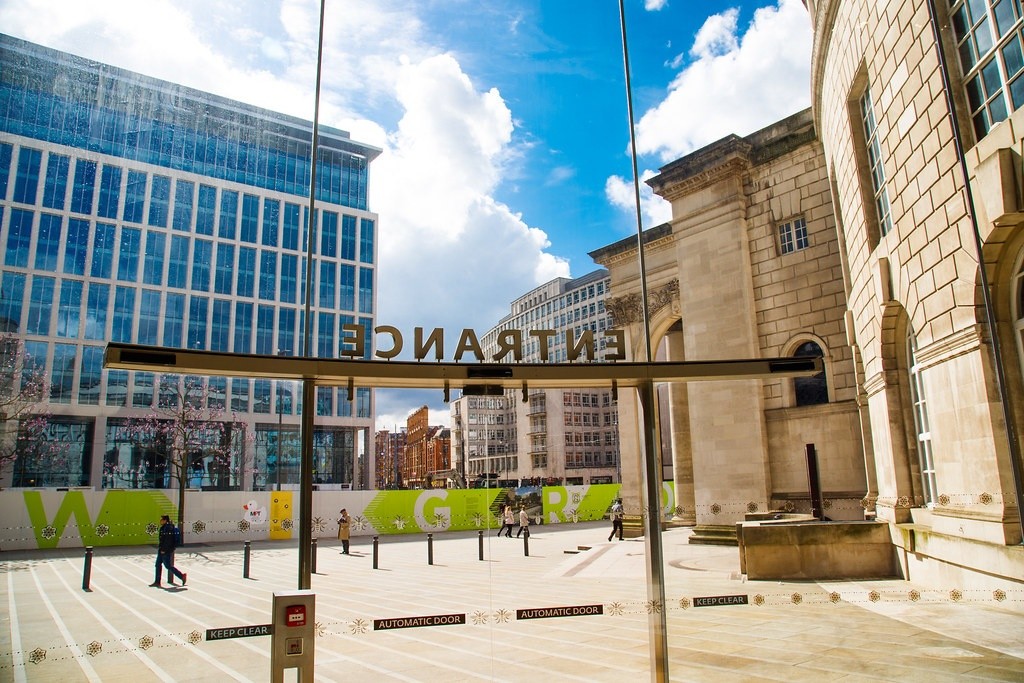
[505,533,508,537]
[517,535,519,538]
[340,551,348,554]
[181,573,186,586]
[608,538,611,542]
[619,538,624,540]
[498,533,499,537]
[148,582,161,587]
[509,535,512,537]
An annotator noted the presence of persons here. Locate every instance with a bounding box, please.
[337,509,351,554]
[608,498,624,542]
[505,506,514,538]
[498,505,509,537]
[148,515,186,587]
[517,505,530,538]
